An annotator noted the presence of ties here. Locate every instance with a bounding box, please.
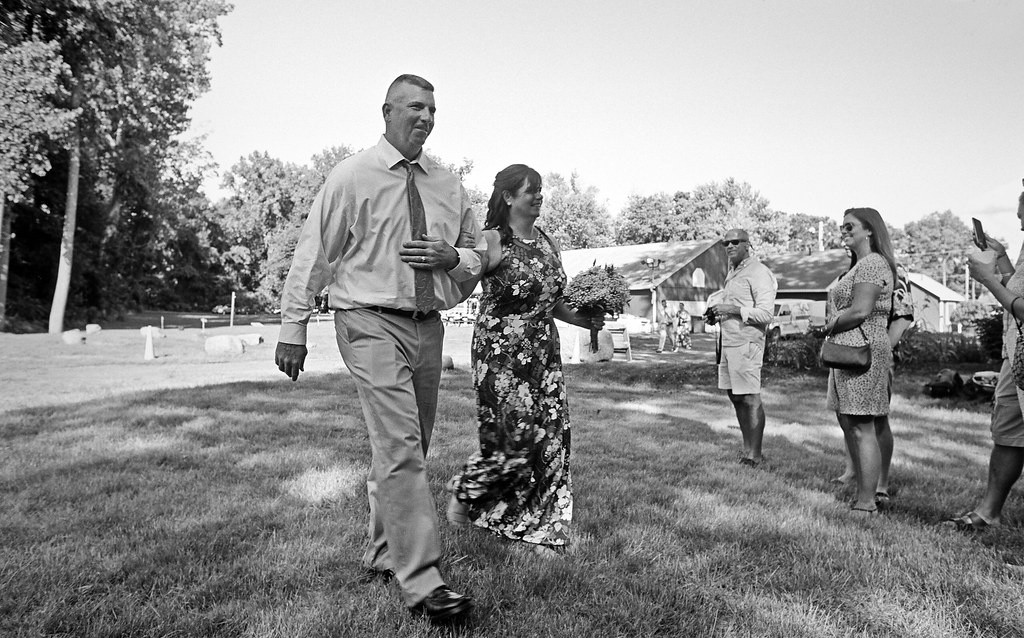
[402,161,436,315]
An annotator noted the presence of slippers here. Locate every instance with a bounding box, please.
[853,507,877,520]
[943,512,1001,531]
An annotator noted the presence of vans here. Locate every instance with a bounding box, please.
[767,302,812,342]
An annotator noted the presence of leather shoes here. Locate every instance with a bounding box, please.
[409,585,470,622]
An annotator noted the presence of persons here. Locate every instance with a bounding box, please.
[275,73,488,619]
[444,163,605,557]
[657,177,1024,533]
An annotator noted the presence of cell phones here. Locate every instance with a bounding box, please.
[972,217,987,248]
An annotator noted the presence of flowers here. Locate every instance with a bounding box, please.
[561,260,631,353]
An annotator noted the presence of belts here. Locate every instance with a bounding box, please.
[365,306,438,321]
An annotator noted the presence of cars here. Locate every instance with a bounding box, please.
[212,303,259,315]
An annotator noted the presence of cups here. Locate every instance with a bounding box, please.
[807,301,826,325]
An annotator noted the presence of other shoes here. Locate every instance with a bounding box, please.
[876,490,890,507]
[448,475,468,529]
[686,345,691,350]
[671,347,678,352]
[738,451,764,469]
[656,350,662,353]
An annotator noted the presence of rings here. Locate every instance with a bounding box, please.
[421,256,426,263]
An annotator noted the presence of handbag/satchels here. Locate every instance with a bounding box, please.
[820,316,872,373]
[1011,297,1024,391]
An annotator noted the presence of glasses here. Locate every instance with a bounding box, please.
[839,223,862,231]
[722,239,746,247]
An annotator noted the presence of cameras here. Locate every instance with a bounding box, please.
[703,307,715,320]
